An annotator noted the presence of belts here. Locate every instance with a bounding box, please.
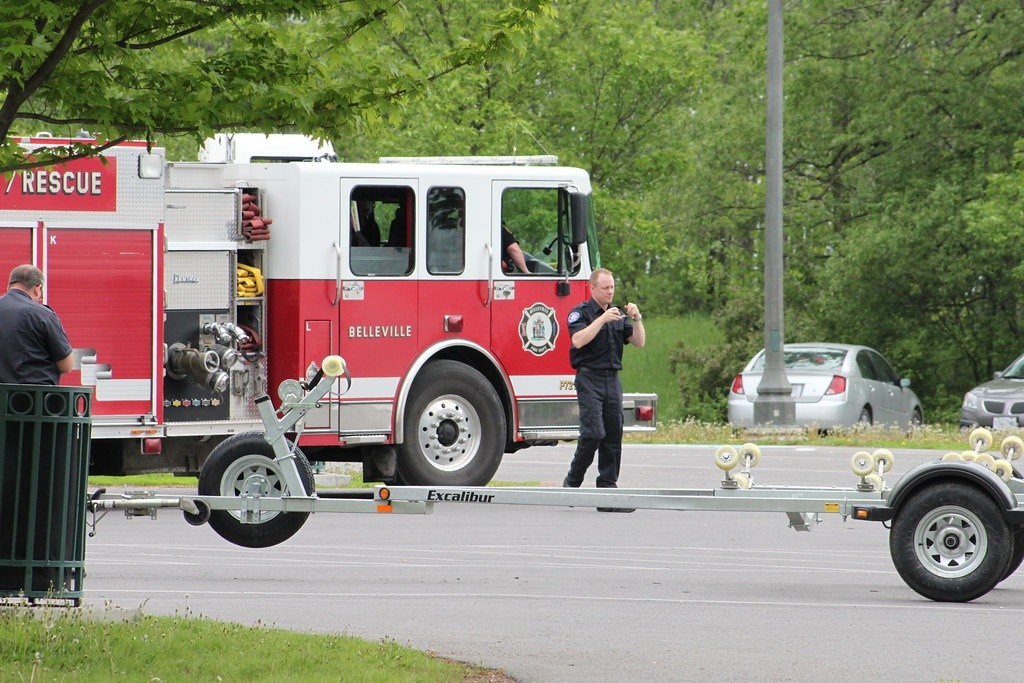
[580,367,618,376]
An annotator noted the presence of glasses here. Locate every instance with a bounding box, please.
[620,297,630,317]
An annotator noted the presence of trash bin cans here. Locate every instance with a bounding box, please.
[0,383,96,606]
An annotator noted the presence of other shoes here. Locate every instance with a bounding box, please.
[597,507,635,513]
[563,478,575,507]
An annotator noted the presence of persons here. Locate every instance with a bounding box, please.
[563,269,645,513]
[1,264,74,385]
[501,220,533,274]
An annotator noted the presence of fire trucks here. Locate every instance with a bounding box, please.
[0,131,658,486]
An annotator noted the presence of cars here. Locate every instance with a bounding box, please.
[959,352,1024,432]
[727,342,924,438]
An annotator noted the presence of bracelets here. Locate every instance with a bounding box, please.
[632,315,643,322]
